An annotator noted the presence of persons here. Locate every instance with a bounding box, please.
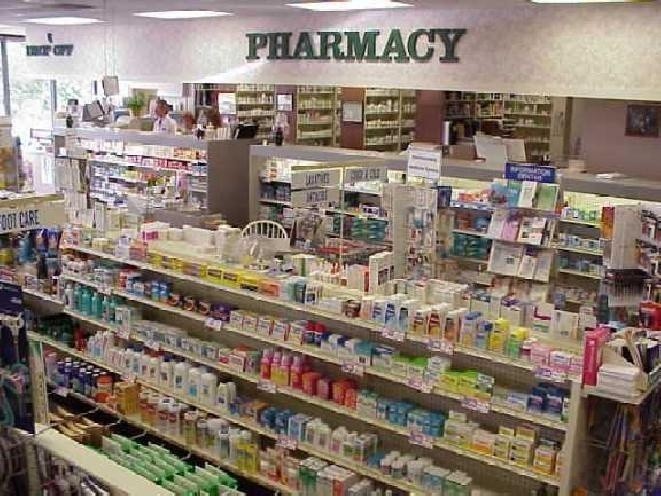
[177,113,194,134]
[152,98,177,131]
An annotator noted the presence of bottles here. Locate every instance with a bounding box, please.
[57,254,302,487]
